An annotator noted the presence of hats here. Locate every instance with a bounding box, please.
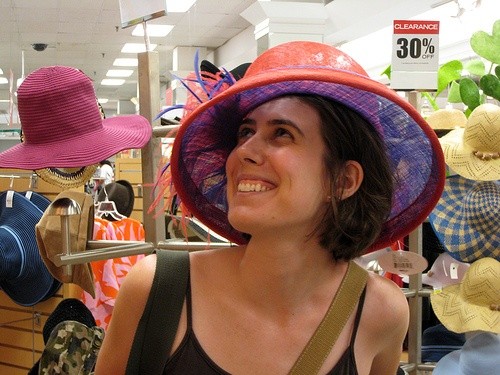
[0,98,230,375]
[170,41,445,260]
[353,102,500,375]
[0,65,151,169]
[200,60,252,88]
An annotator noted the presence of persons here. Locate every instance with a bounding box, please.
[94,42,446,375]
